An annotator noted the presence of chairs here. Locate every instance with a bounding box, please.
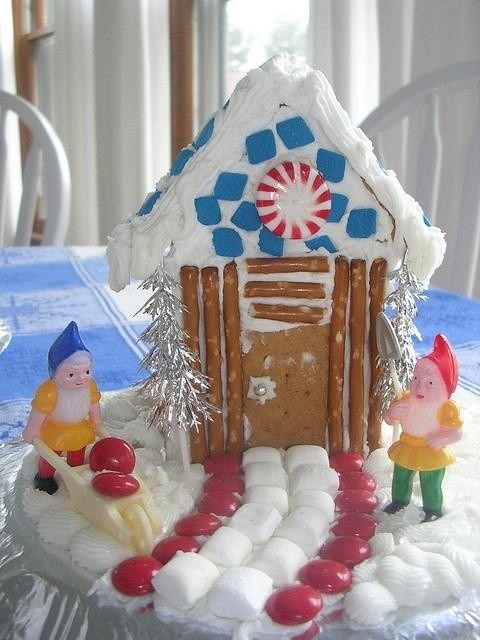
[0,92,71,250]
[358,60,480,302]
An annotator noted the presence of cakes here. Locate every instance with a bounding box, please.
[0,50,480,640]
